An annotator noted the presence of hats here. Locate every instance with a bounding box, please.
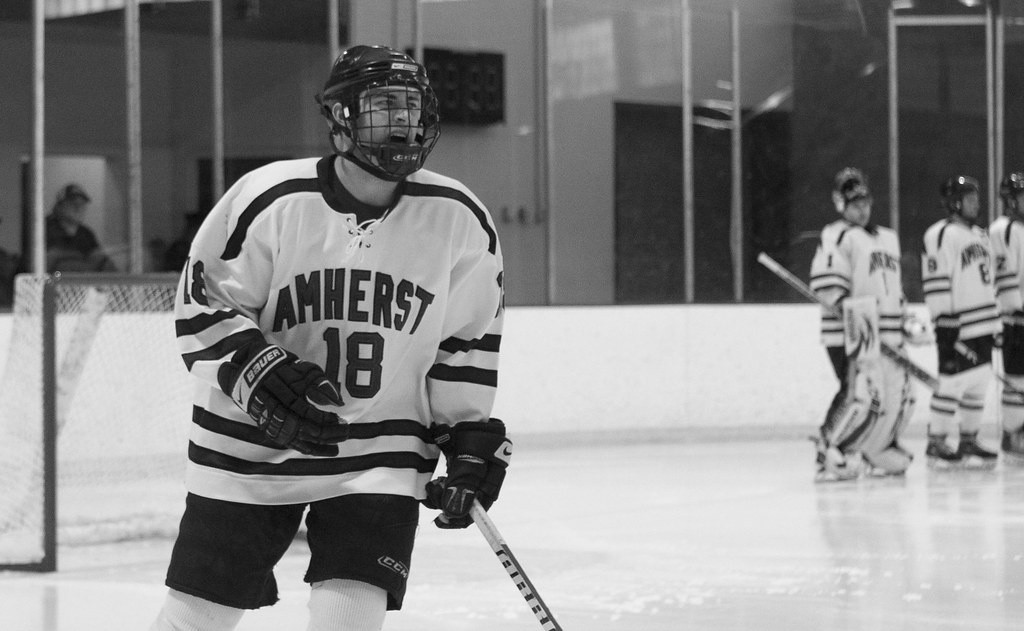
[55,185,91,204]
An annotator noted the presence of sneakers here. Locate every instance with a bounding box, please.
[926,423,998,472]
[1000,420,1024,466]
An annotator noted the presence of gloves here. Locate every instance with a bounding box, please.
[934,314,961,360]
[218,339,347,456]
[421,418,513,529]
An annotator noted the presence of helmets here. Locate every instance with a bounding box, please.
[1000,171,1024,196]
[314,45,441,182]
[940,175,979,221]
[831,168,873,213]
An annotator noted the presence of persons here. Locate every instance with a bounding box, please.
[19,183,120,274]
[921,171,1024,471]
[151,44,513,631]
[809,166,926,483]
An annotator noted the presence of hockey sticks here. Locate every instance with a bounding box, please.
[756,248,941,389]
[463,493,565,631]
[954,341,1024,400]
